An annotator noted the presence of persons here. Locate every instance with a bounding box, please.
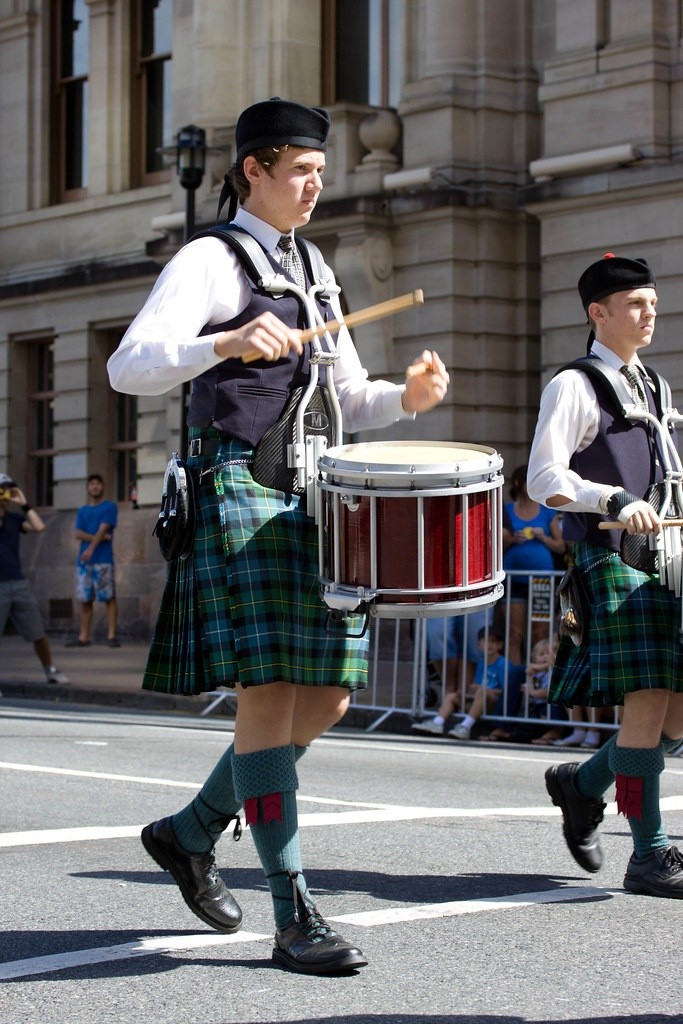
[107,95,452,974]
[0,472,70,686]
[527,253,683,900]
[410,463,624,748]
[64,473,121,648]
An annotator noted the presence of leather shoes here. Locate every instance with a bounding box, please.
[623,846,683,899]
[272,911,368,974]
[140,815,242,934]
[545,762,607,873]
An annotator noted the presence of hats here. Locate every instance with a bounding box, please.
[0,473,17,488]
[216,97,330,226]
[578,252,656,310]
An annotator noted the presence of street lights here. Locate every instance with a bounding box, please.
[175,124,208,464]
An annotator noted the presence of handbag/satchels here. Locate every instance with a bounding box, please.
[156,452,195,560]
[556,564,589,650]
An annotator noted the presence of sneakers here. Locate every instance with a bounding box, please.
[553,737,599,747]
[411,719,443,734]
[44,665,68,685]
[448,724,470,740]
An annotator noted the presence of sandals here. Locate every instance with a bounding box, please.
[480,729,516,741]
[531,734,561,746]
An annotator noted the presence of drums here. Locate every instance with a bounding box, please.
[317,440,508,618]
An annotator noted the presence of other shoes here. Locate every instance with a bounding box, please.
[64,638,91,648]
[105,636,121,647]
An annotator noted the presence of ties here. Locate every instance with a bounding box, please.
[277,236,306,295]
[619,365,649,425]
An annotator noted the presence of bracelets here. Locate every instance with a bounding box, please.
[21,504,32,512]
